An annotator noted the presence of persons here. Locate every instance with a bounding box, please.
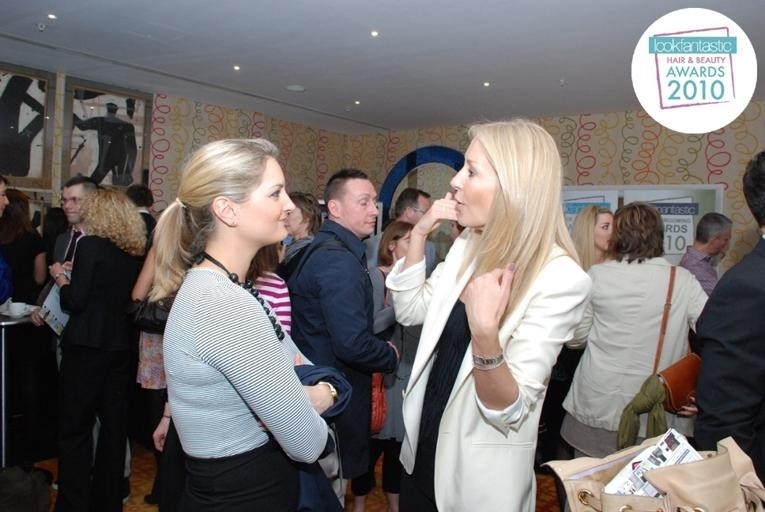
[0,116,765,511]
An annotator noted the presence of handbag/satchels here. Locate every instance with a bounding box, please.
[655,352,704,415]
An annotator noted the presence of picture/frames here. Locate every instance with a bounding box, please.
[60,75,153,192]
[561,183,723,285]
[0,60,58,192]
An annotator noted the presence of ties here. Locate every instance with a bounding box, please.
[47,229,83,291]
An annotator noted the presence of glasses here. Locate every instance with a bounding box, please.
[412,207,429,215]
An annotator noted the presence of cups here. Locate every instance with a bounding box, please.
[8,303,28,315]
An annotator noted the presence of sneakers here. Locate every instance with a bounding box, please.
[51,479,59,492]
[123,479,133,506]
